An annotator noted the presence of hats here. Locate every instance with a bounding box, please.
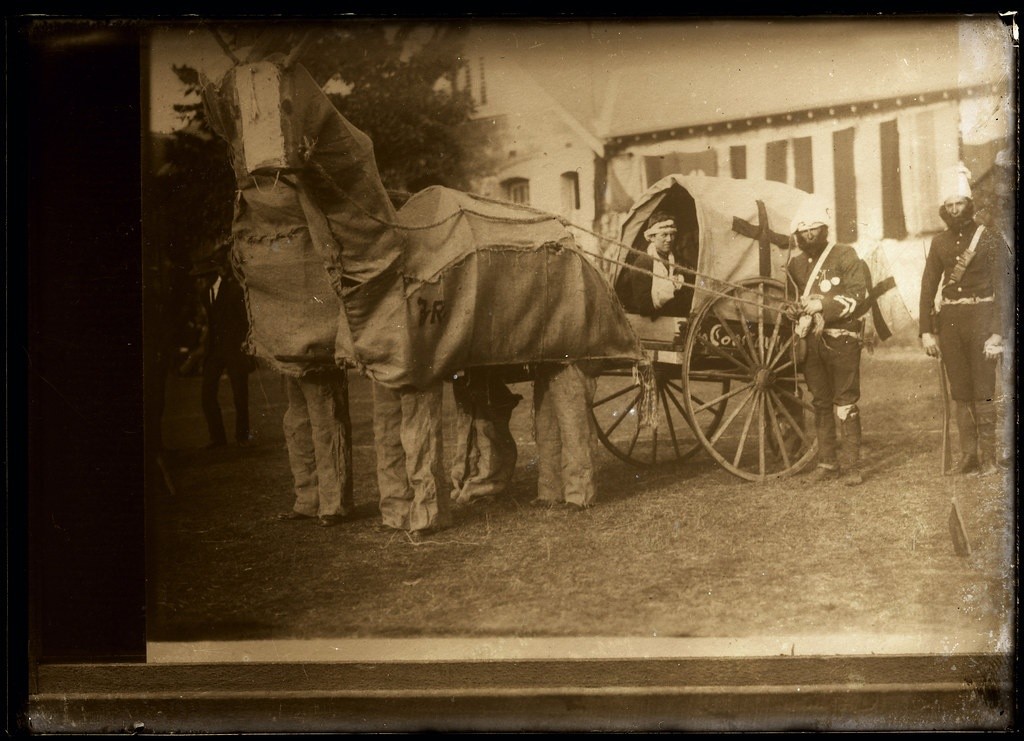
[790,194,829,237]
[937,155,972,207]
[188,259,220,277]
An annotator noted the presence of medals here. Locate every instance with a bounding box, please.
[818,275,840,293]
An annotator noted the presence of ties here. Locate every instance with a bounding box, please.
[210,286,216,306]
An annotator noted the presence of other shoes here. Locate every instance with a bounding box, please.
[843,458,865,485]
[945,450,978,476]
[977,451,998,476]
[233,433,250,448]
[198,437,226,449]
[801,459,840,489]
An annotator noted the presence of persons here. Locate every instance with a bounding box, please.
[277,369,598,541]
[615,210,696,318]
[193,263,253,450]
[918,171,1014,476]
[785,199,872,487]
[143,255,175,463]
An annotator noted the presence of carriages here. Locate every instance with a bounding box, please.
[194,0,833,537]
